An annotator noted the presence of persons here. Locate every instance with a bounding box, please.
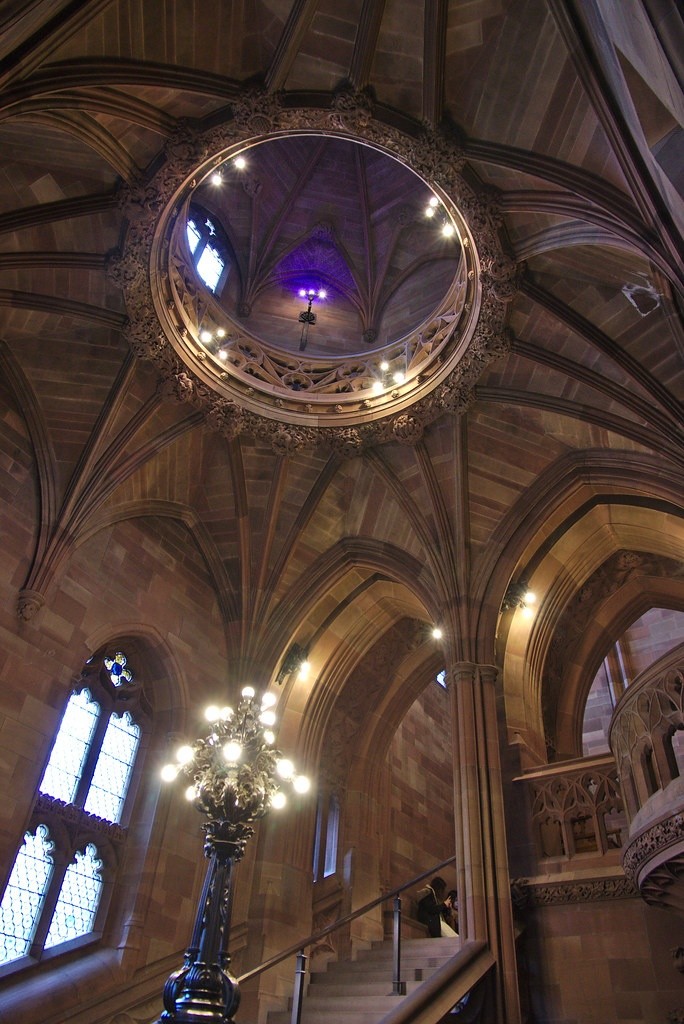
[418,877,451,938]
[445,890,459,933]
